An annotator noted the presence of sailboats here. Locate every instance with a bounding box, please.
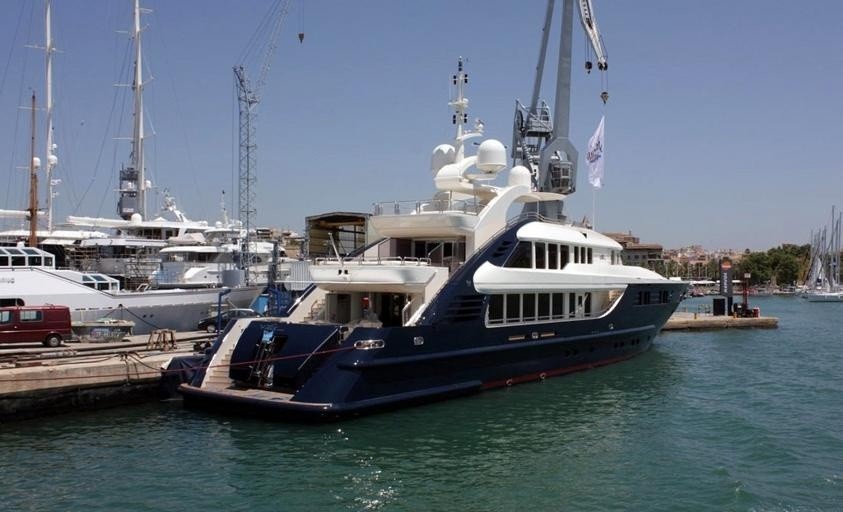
[795,203,842,301]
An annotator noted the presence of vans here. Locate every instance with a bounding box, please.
[1,304,74,348]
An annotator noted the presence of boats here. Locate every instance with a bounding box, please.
[685,285,794,299]
[179,0,687,418]
[0,1,307,334]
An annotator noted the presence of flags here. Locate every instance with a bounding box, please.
[587,117,603,188]
